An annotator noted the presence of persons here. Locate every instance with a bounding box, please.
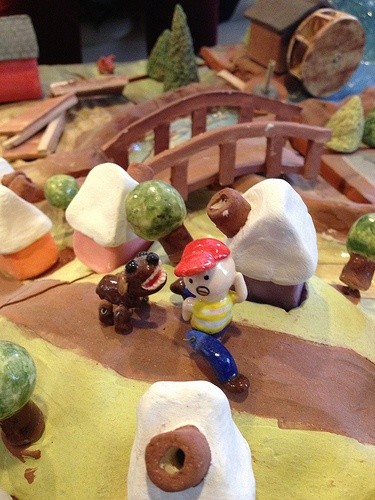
[173,236,249,393]
